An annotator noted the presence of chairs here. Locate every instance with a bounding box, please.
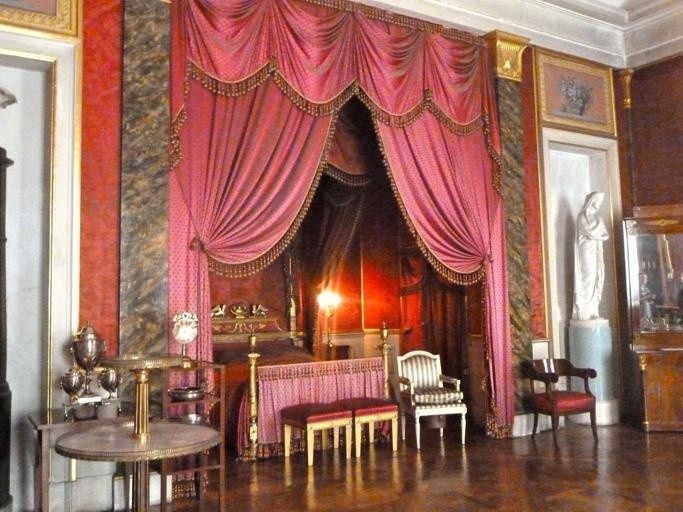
[390,350,467,449]
[521,359,598,442]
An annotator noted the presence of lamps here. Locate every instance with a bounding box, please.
[59,320,122,423]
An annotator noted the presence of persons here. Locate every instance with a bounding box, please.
[572,191,610,320]
[639,273,656,325]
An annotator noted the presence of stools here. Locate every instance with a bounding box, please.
[333,398,398,458]
[280,403,353,465]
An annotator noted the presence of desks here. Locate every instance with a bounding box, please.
[55,422,222,512]
[27,401,156,512]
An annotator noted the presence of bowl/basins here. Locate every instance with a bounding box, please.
[168,386,204,401]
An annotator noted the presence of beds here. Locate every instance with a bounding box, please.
[210,304,390,461]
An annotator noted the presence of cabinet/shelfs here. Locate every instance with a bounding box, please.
[126,360,226,512]
[622,204,683,433]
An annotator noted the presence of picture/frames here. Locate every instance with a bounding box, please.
[534,46,618,139]
[0,0,80,37]
